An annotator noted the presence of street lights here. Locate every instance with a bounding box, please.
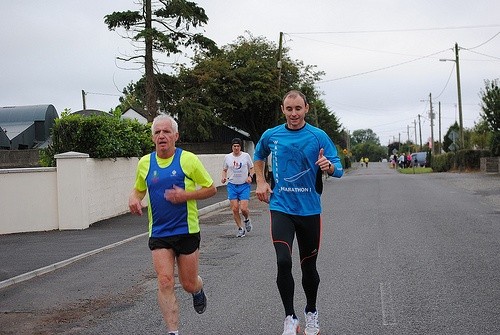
[439,43,464,149]
[386,114,422,152]
[419,93,434,154]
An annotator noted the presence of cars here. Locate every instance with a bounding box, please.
[411,152,426,166]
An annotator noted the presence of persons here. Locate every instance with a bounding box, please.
[253,89,344,335]
[360,156,369,168]
[390,153,418,169]
[221,138,255,237]
[128,115,218,335]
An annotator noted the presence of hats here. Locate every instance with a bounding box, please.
[231,138,242,147]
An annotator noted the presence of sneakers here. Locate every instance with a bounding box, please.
[282,315,301,335]
[244,217,251,232]
[303,307,321,335]
[192,287,207,314]
[236,227,245,237]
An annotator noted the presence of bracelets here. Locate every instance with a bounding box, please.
[329,161,332,170]
[249,174,253,178]
